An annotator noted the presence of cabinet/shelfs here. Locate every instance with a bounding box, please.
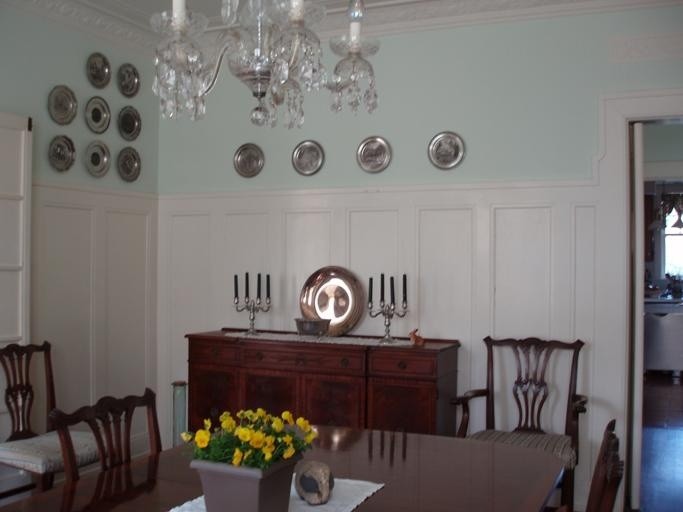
[186,327,304,442]
[305,340,460,441]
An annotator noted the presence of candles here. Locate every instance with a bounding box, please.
[368,278,373,310]
[266,275,270,297]
[403,275,407,302]
[246,273,249,298]
[234,274,238,297]
[380,275,385,302]
[390,278,394,304]
[258,274,261,299]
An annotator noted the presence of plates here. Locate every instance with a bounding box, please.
[83,94,111,134]
[232,142,265,179]
[291,140,325,176]
[115,146,143,183]
[115,104,143,141]
[426,131,465,169]
[355,136,390,173]
[298,266,364,338]
[46,84,77,124]
[85,50,112,88]
[47,134,75,172]
[82,139,111,178]
[115,62,141,97]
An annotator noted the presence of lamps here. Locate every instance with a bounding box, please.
[144,1,378,130]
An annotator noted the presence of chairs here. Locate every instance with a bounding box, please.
[51,389,162,480]
[1,341,114,494]
[451,337,586,475]
[549,419,626,511]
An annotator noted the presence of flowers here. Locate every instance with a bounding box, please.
[177,406,317,469]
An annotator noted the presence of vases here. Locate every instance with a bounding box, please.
[188,457,303,510]
